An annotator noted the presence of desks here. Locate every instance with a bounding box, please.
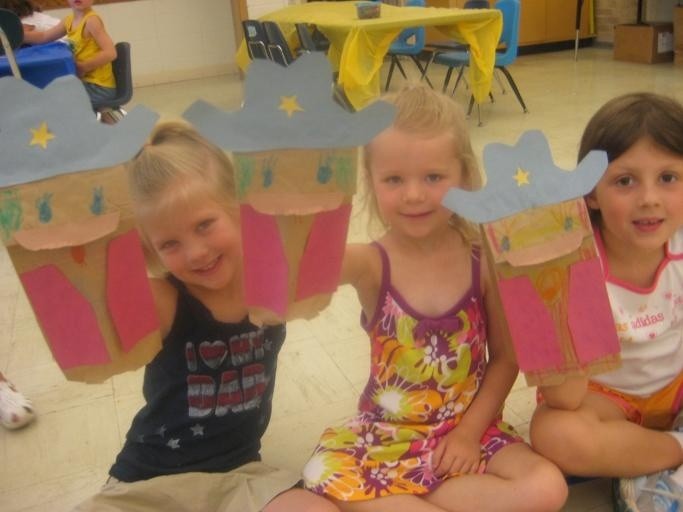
[1,37,74,89]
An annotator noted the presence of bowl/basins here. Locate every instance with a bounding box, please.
[354,3,380,19]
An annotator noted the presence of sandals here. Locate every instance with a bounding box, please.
[612,475,645,511]
[1,373,36,429]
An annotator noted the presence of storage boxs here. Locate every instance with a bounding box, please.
[613,22,674,66]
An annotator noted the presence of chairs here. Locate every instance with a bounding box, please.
[94,40,135,125]
[0,8,24,54]
[240,1,529,128]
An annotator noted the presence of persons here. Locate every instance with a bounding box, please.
[21,1,117,120]
[526,93,683,511]
[70,119,345,511]
[299,84,570,511]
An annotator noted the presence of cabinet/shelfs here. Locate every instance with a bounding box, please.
[424,0,598,52]
